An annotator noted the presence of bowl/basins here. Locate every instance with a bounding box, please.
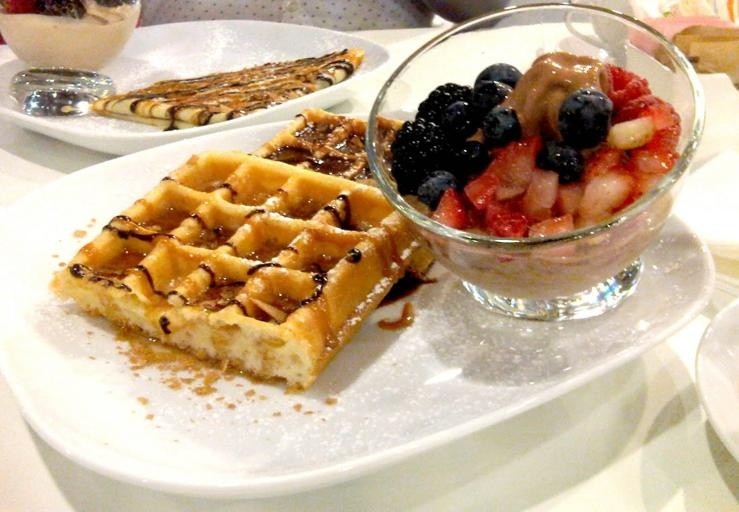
[1,1,142,71]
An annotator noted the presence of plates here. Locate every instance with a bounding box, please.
[5,120,719,498]
[0,18,388,154]
[695,296,739,456]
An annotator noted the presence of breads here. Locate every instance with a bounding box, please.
[52,106,434,389]
[654,23,739,84]
[90,46,366,131]
